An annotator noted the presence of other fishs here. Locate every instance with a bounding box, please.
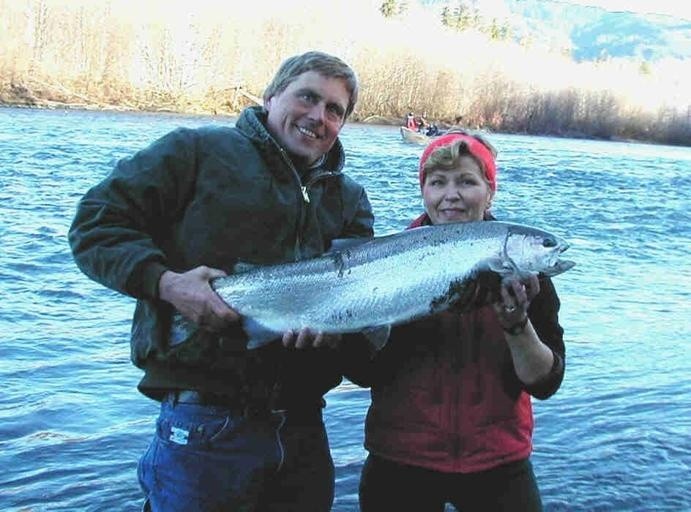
[164,220,577,350]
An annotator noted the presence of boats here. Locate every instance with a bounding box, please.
[400,126,439,147]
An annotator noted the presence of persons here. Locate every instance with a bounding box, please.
[280,127,568,512]
[67,48,373,511]
[404,110,464,138]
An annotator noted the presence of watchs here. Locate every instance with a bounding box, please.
[505,314,528,336]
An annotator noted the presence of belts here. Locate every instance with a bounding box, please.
[175,391,209,407]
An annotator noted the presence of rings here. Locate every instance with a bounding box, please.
[505,307,517,314]
